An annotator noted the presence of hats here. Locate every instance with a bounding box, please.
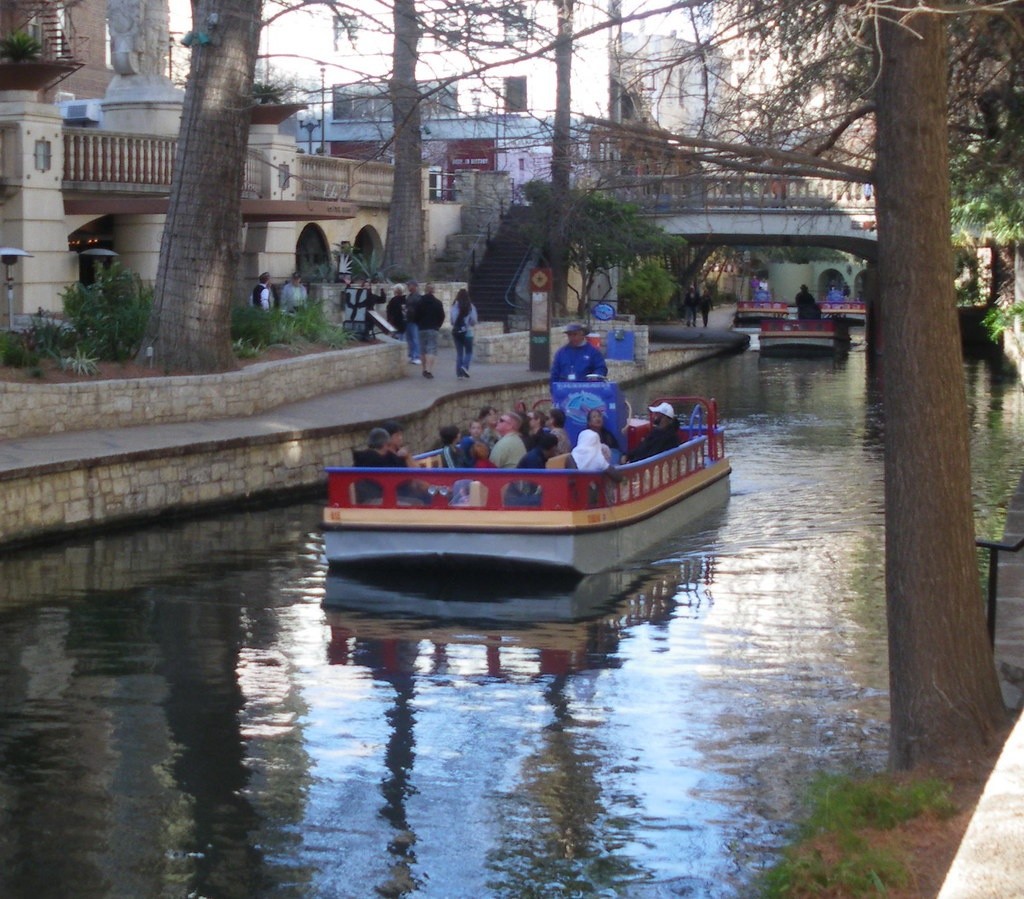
[563,324,590,336]
[292,273,303,278]
[649,403,674,418]
[405,279,418,286]
[800,284,808,288]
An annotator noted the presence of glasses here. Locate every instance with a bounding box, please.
[499,419,512,426]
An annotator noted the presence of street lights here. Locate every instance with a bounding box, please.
[297,108,322,154]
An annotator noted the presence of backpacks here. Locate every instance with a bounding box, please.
[453,314,467,339]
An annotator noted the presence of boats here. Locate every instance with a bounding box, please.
[736,291,788,326]
[815,291,867,326]
[758,313,851,355]
[322,381,733,575]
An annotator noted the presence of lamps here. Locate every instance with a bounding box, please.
[80,248,120,295]
[0,247,35,330]
[180,30,209,48]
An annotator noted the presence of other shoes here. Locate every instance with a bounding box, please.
[456,366,470,379]
[421,369,434,379]
[409,358,423,364]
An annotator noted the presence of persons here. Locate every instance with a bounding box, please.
[548,323,607,401]
[795,284,821,321]
[340,274,424,365]
[751,275,768,302]
[351,420,431,506]
[451,288,478,380]
[249,271,308,317]
[416,282,446,379]
[440,403,682,508]
[683,283,715,328]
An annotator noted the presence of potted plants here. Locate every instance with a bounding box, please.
[253,82,310,125]
[0,30,75,90]
